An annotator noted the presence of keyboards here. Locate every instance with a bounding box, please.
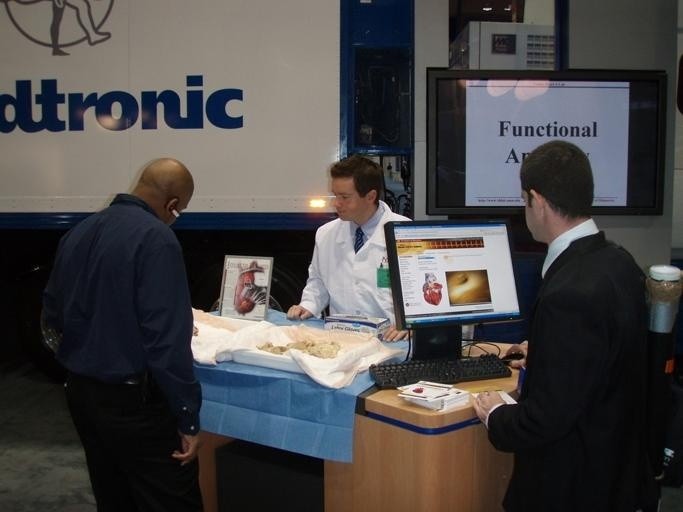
[369,353,513,390]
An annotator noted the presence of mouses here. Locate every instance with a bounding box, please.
[502,353,525,365]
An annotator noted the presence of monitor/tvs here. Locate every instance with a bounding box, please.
[425,66,668,216]
[383,218,528,360]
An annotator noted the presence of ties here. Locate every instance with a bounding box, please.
[353,227,365,254]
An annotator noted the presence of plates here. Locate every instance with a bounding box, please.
[231,351,306,375]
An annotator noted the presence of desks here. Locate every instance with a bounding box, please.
[191,306,531,512]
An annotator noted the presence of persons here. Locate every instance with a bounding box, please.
[36,156,205,512]
[468,136,664,512]
[286,150,415,342]
